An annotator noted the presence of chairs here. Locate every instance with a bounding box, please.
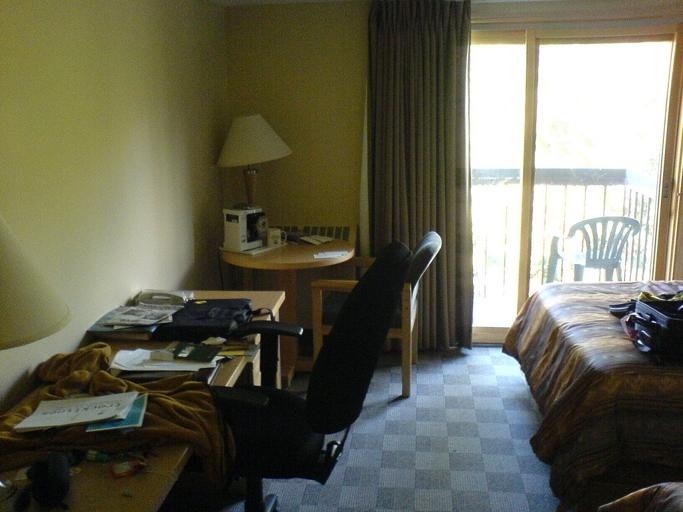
[546,213,638,284]
[186,243,408,511]
[303,232,444,397]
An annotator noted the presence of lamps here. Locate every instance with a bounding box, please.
[215,113,292,206]
[2,217,73,350]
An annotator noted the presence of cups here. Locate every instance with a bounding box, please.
[29,450,70,505]
[266,228,287,248]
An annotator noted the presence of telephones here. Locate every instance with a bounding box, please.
[134,289,194,304]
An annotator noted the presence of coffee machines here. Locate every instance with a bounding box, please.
[220,206,264,252]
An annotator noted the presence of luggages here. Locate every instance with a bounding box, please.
[608,293,683,357]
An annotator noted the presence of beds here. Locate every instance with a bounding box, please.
[499,283,683,463]
[598,482,682,510]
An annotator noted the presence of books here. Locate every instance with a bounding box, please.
[86,305,172,341]
[111,314,172,330]
[171,338,226,365]
[12,390,138,433]
[84,392,148,433]
[96,303,185,324]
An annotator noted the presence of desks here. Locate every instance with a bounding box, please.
[0,286,284,510]
[220,238,353,387]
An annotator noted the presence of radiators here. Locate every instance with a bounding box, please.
[260,222,357,253]
